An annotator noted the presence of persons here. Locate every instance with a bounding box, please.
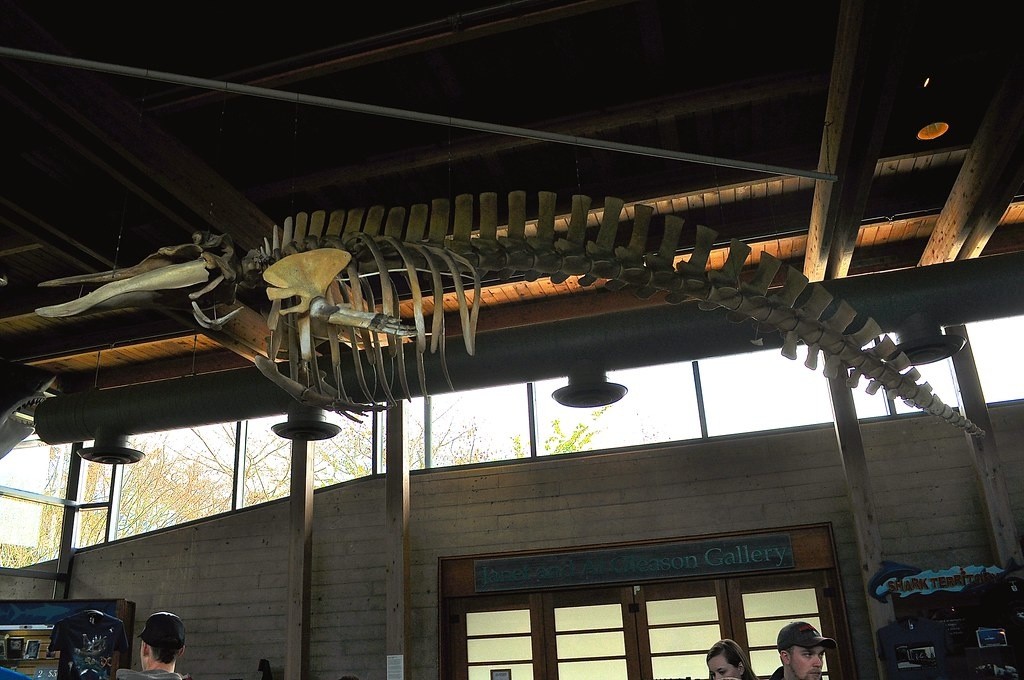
[706,638,760,680]
[114,611,186,680]
[767,622,838,680]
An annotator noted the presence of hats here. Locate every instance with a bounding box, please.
[136,611,185,650]
[777,621,837,652]
